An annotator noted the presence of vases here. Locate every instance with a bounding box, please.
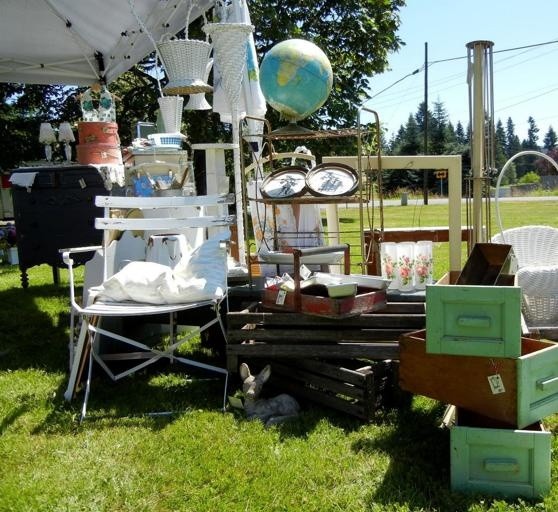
[7,247,19,265]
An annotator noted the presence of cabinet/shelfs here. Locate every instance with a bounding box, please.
[240,106,385,289]
[12,163,125,289]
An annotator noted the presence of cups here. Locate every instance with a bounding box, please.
[378,239,434,291]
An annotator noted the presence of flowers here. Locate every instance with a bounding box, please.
[0,223,17,247]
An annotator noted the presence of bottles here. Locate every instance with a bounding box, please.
[179,161,197,197]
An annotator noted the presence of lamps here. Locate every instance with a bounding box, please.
[39,121,75,164]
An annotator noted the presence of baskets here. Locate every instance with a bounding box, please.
[157,33,214,95]
[184,4,212,111]
[157,96,185,133]
[486,151,558,339]
[201,22,253,101]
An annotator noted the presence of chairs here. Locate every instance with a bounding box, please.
[60,194,237,423]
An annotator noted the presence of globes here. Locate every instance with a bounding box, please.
[259,39,333,134]
